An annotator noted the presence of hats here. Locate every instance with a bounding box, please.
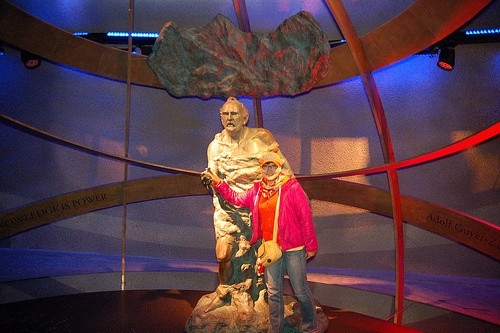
[260,153,281,167]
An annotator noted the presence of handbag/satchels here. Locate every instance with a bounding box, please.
[258,240,282,267]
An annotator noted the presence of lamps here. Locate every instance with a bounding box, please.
[436,44,456,71]
[20,51,41,69]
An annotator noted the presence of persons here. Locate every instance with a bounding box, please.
[204,153,319,333]
[200,96,297,312]
